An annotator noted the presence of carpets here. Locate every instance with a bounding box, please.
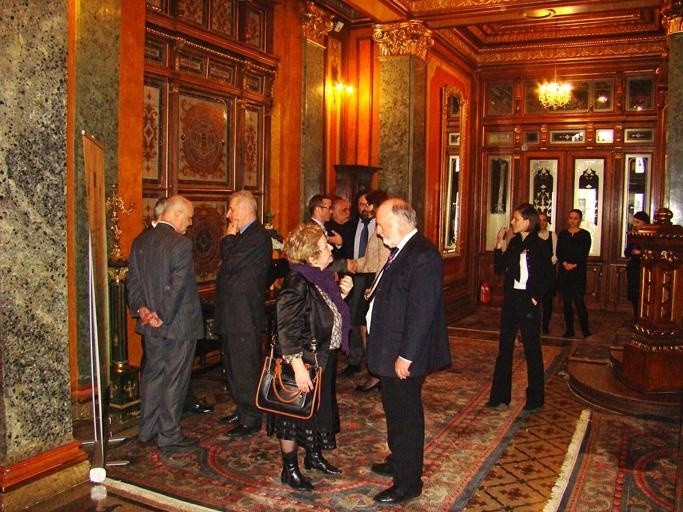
[90,328,574,512]
[543,409,683,512]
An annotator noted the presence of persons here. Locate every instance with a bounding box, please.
[624,211,650,329]
[485,203,555,409]
[264,224,354,491]
[124,195,201,448]
[536,212,559,335]
[145,198,215,415]
[365,196,452,505]
[307,189,393,395]
[556,209,593,337]
[211,190,273,437]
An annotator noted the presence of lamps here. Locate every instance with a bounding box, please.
[536,18,575,109]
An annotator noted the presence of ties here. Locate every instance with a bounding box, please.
[358,219,368,258]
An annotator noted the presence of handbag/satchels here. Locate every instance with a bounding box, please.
[255,356,322,420]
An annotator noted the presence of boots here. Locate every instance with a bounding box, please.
[281,451,314,491]
[305,447,342,476]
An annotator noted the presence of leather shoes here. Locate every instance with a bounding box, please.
[371,462,393,478]
[523,400,545,412]
[561,332,576,337]
[354,379,381,394]
[219,415,240,425]
[226,425,261,438]
[374,485,422,505]
[342,364,361,376]
[485,399,511,408]
[188,402,214,414]
[170,435,199,448]
